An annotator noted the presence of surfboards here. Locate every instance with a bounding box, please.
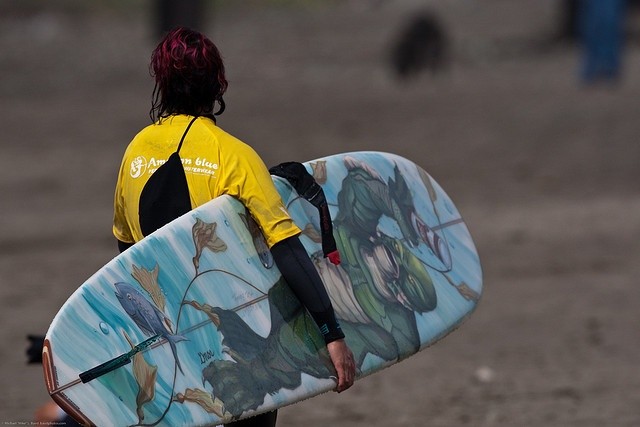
[42,150,483,427]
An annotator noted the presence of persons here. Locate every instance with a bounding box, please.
[31,396,81,426]
[113,29,355,427]
